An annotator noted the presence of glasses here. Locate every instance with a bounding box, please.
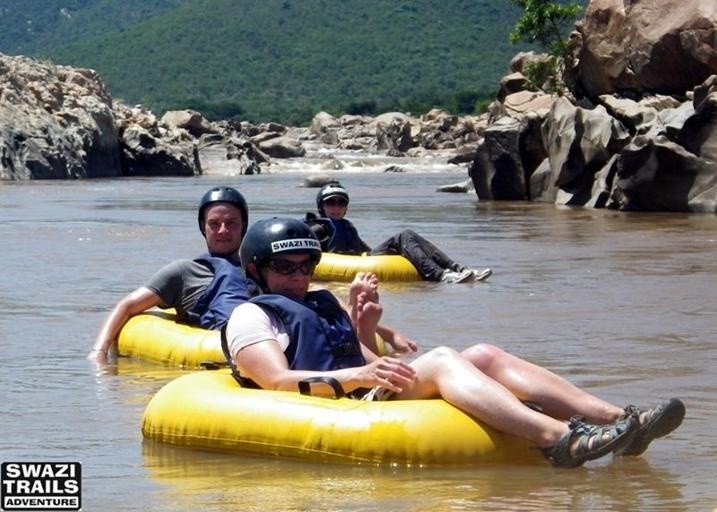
[262,256,316,275]
[323,198,347,207]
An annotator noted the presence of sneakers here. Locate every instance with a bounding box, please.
[440,268,472,283]
[462,267,492,281]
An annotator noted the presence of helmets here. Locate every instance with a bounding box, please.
[198,185,248,237]
[317,182,349,218]
[239,217,322,279]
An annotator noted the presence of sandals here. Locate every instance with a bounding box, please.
[541,412,635,468]
[615,398,686,455]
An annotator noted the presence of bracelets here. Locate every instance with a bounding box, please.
[90,348,108,356]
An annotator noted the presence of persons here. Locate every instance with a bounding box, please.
[87,186,259,365]
[299,183,492,285]
[221,217,685,467]
